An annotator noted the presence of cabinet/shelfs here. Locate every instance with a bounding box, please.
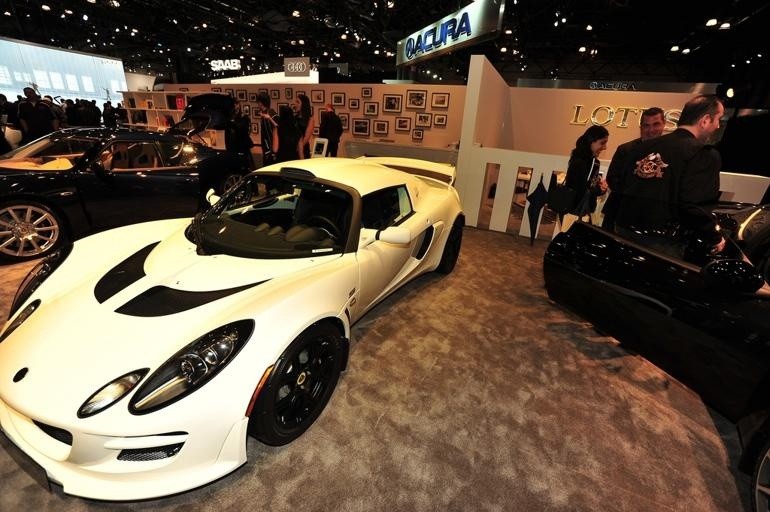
[118,90,199,133]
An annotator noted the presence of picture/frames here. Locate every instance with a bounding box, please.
[211,86,451,142]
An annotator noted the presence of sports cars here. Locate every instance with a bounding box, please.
[1,157,464,499]
[0,95,255,256]
[541,197,770,512]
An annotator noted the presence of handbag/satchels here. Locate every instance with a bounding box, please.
[548,184,579,215]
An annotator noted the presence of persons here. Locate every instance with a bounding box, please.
[0,86,127,156]
[602,107,667,228]
[610,92,728,263]
[550,125,608,243]
[223,93,343,198]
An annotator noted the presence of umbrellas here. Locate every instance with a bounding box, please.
[526,171,548,243]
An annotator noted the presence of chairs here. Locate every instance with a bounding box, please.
[104,144,157,170]
